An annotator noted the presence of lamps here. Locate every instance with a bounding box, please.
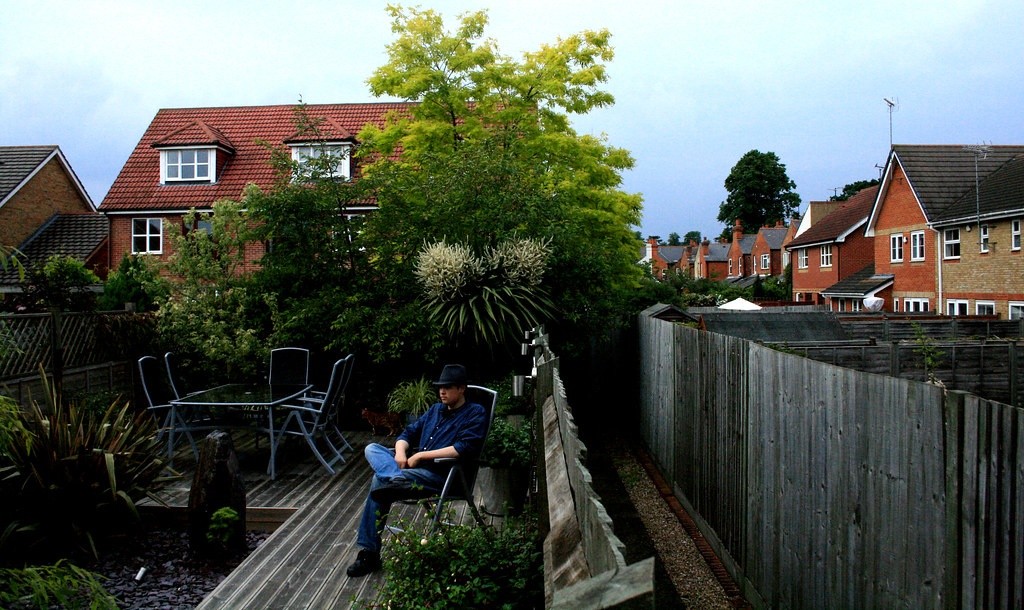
[521,343,532,356]
[511,374,532,398]
[524,331,534,339]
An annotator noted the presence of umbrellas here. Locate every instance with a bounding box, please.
[718,297,762,313]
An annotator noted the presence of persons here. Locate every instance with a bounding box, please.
[346,364,486,577]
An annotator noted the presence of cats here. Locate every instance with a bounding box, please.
[361,407,406,436]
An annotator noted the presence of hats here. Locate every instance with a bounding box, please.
[433,364,469,385]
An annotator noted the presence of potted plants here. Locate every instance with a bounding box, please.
[477,416,536,517]
[486,369,532,427]
[387,374,441,425]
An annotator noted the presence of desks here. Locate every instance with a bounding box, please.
[168,382,314,481]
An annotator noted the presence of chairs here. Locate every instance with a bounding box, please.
[251,358,346,475]
[136,355,231,465]
[384,385,498,539]
[254,346,310,449]
[164,351,216,422]
[280,354,355,463]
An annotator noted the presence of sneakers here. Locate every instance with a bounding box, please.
[347,550,380,577]
[370,479,410,504]
